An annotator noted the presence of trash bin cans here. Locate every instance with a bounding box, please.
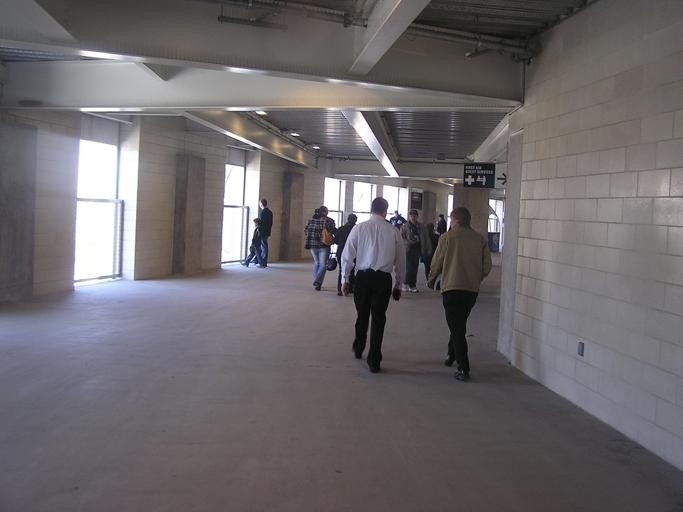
[488,232,500,252]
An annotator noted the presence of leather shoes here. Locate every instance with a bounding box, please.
[443,354,455,366]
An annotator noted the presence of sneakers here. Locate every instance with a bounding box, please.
[400,285,419,293]
[312,280,321,291]
[239,261,268,269]
[454,370,469,381]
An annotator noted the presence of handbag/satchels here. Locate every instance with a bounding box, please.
[319,216,334,246]
[326,248,338,272]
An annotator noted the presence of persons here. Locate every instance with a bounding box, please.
[302,198,492,381]
[240,217,267,267]
[257,196,274,261]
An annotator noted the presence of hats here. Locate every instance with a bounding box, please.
[407,209,418,217]
